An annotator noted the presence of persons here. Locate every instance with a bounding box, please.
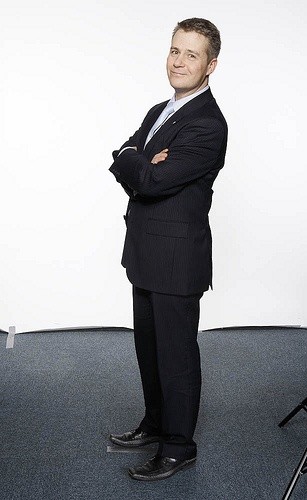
[109,18,227,480]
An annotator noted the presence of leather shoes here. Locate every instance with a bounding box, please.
[128,453,197,481]
[109,428,159,447]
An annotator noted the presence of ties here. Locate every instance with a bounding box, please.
[147,105,173,138]
